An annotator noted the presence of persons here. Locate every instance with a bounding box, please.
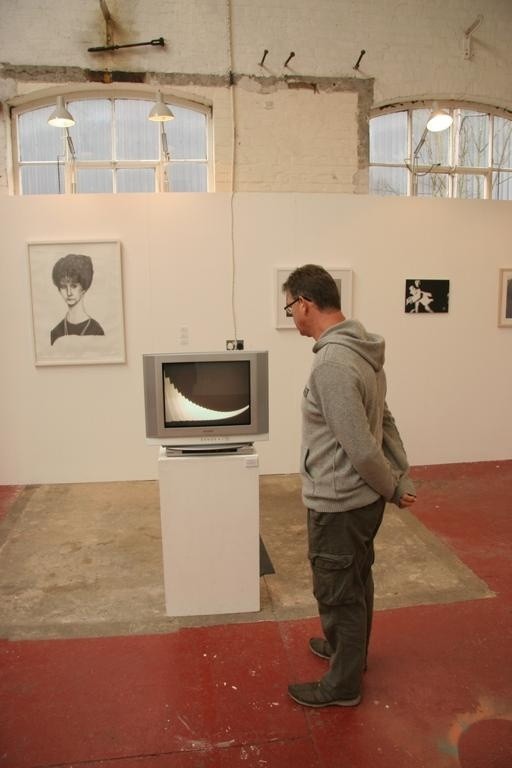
[51,254,104,345]
[284,264,416,707]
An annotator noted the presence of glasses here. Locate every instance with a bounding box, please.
[284,296,311,313]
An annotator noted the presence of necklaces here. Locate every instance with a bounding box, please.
[64,311,90,335]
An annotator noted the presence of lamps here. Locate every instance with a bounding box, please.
[48,96,79,194]
[405,107,453,195]
[148,92,175,192]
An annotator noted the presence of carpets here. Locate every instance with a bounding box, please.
[1,473,500,639]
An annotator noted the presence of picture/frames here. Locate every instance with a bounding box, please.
[273,265,353,330]
[497,267,512,328]
[24,235,129,370]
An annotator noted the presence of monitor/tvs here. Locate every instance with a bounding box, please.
[143,351,270,457]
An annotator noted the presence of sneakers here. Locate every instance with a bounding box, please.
[288,681,360,707]
[309,637,335,658]
